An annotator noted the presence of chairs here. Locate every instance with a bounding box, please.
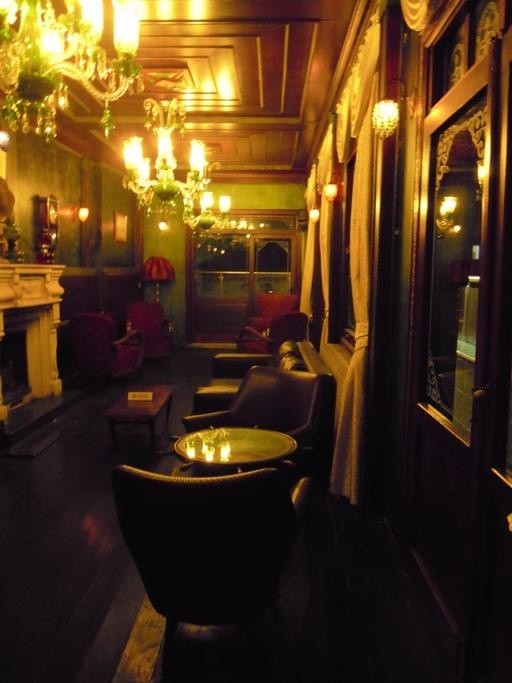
[74,312,142,383]
[250,295,298,329]
[125,301,172,366]
[182,367,323,466]
[237,312,306,350]
[112,465,336,627]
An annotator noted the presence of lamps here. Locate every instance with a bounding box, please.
[308,209,322,225]
[0,0,139,144]
[323,181,338,202]
[142,256,176,320]
[122,99,231,232]
[79,205,90,222]
[371,101,401,137]
[436,199,463,236]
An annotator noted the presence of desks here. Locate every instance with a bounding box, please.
[107,385,175,447]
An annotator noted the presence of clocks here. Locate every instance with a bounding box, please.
[32,194,58,264]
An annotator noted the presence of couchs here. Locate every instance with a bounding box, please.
[194,339,331,476]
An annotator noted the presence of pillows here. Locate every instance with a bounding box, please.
[278,353,304,370]
[276,340,298,361]
